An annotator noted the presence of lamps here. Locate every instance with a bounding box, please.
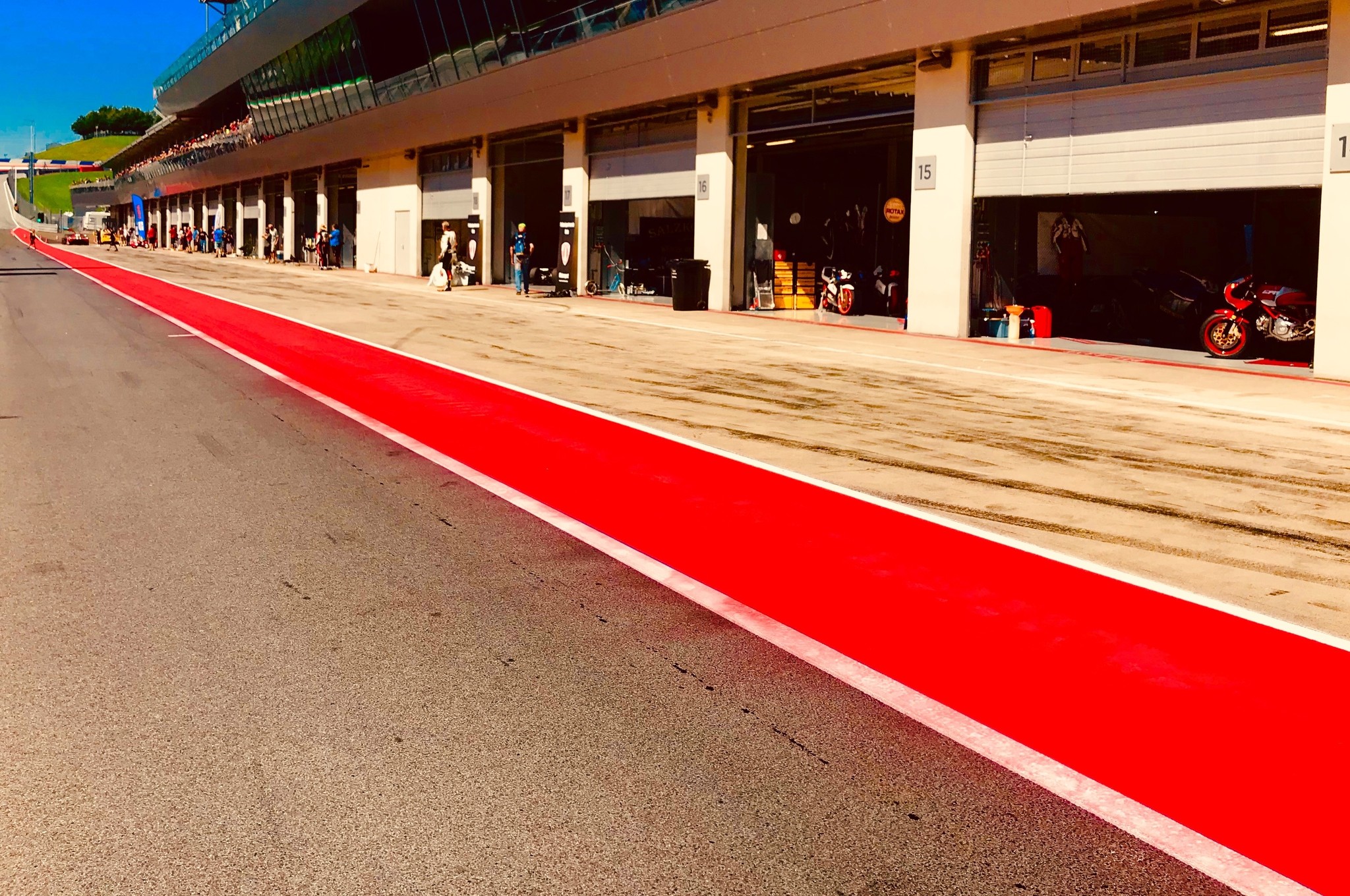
[562,123,578,133]
[918,49,952,71]
[696,94,718,111]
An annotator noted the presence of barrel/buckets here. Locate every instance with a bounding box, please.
[1031,306,1052,338]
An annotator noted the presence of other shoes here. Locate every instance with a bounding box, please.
[516,292,521,295]
[524,292,528,295]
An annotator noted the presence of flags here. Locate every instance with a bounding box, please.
[131,194,147,241]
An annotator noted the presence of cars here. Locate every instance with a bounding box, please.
[66,234,89,245]
[95,229,120,245]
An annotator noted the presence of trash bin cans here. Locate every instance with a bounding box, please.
[666,258,709,311]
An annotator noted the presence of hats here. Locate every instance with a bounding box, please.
[319,224,327,231]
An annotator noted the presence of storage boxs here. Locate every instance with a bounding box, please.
[985,318,1030,339]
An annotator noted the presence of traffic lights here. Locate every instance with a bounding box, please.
[37,212,44,223]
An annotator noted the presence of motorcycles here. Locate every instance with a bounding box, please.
[821,265,856,316]
[1197,274,1317,369]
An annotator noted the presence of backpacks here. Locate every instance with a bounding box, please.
[322,232,329,243]
[514,232,530,264]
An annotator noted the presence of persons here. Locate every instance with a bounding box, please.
[106,228,120,252]
[315,222,342,270]
[118,225,133,247]
[94,228,102,244]
[509,222,535,295]
[168,225,235,258]
[437,220,459,293]
[26,229,37,250]
[14,204,19,213]
[148,224,158,251]
[72,113,275,195]
[262,224,279,264]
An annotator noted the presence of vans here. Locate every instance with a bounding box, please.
[81,211,111,232]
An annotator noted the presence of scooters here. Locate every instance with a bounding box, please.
[584,260,626,297]
